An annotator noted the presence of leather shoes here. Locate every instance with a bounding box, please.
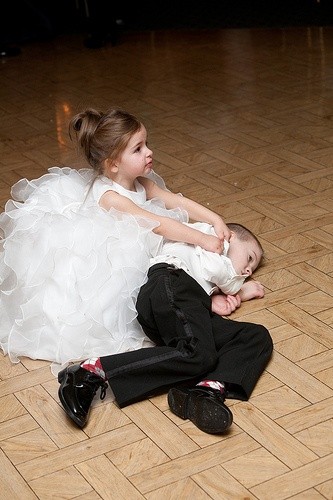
[168,382,233,433]
[58,363,105,426]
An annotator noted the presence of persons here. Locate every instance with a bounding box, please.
[58,223,274,434]
[0,107,232,379]
